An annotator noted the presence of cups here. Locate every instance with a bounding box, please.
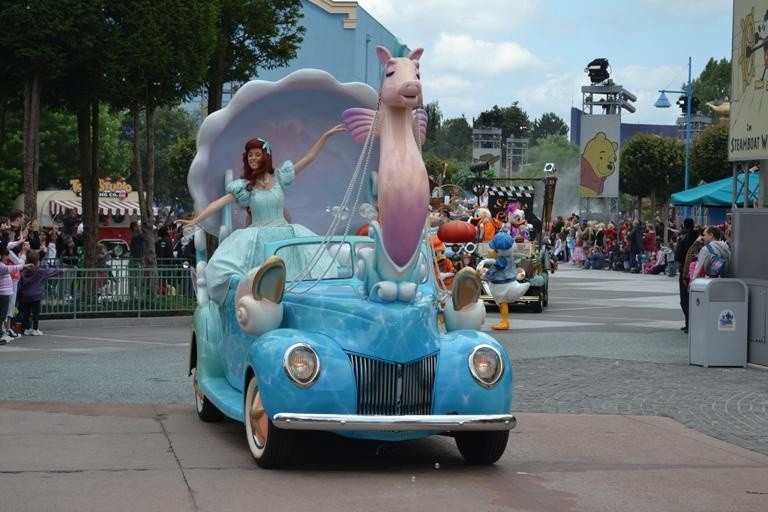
[15,322,22,333]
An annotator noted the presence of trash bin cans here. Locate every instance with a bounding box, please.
[689,278,748,368]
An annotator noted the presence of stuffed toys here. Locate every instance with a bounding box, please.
[429,201,533,330]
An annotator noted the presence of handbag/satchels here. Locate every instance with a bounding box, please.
[703,256,725,276]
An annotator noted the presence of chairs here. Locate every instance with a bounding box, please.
[246,204,291,228]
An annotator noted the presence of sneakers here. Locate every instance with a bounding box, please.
[0,328,44,343]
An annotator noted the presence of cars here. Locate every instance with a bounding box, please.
[187,236,516,469]
[478,254,550,312]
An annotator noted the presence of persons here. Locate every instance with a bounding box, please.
[0,207,196,345]
[550,212,732,334]
[173,122,349,308]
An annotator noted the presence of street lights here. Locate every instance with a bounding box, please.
[655,56,691,190]
